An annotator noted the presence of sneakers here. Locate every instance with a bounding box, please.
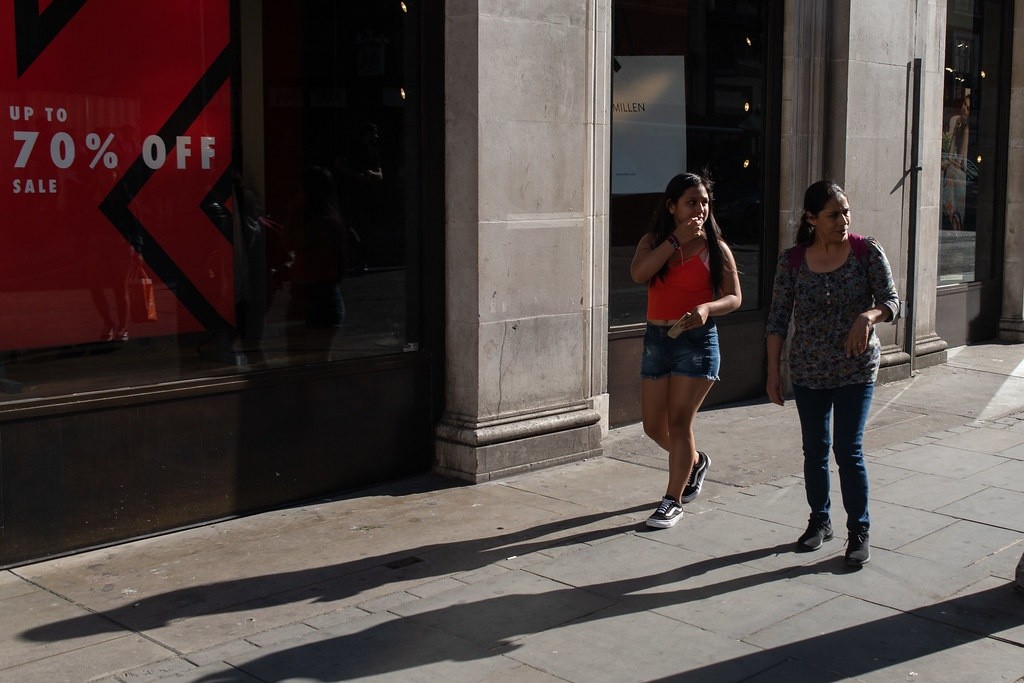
[646,496,684,528]
[796,513,834,552]
[680,451,710,503]
[841,527,871,566]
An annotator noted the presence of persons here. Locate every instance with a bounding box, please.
[630,172,743,529]
[769,180,899,561]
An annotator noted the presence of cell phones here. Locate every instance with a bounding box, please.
[667,312,691,338]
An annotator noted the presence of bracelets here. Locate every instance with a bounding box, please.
[665,233,681,251]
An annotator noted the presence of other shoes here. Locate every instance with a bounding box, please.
[116,327,129,343]
[101,327,114,341]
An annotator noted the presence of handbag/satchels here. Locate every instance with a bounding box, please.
[128,267,158,324]
